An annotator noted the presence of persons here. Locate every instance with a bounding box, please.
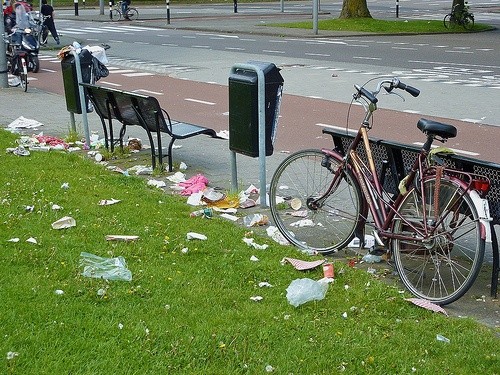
[0,0,60,50]
[121,0,131,19]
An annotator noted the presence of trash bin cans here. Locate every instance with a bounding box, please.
[228,60,284,158]
[61,44,110,114]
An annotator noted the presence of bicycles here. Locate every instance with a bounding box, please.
[109,1,138,21]
[444,4,475,31]
[269,76,493,306]
[28,12,49,45]
[2,34,30,92]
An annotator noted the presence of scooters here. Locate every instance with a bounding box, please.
[11,23,40,73]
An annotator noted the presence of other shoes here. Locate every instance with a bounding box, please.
[56,40,60,44]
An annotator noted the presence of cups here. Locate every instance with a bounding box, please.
[288,198,302,210]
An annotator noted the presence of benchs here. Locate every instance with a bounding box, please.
[78,82,217,172]
[321,127,500,298]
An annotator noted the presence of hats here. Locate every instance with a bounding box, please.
[15,4,21,8]
